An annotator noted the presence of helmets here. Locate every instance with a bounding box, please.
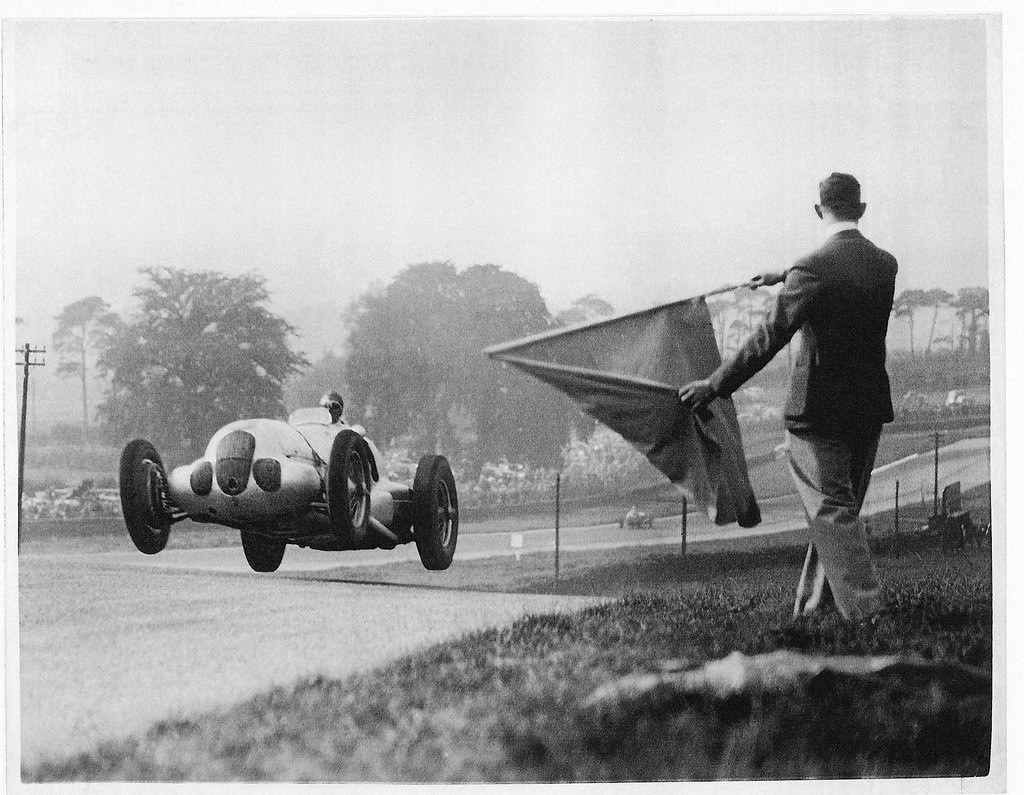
[320,390,343,411]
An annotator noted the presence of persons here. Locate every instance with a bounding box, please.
[679,173,898,626]
[317,389,351,429]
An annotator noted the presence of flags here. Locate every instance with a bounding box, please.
[482,292,761,528]
